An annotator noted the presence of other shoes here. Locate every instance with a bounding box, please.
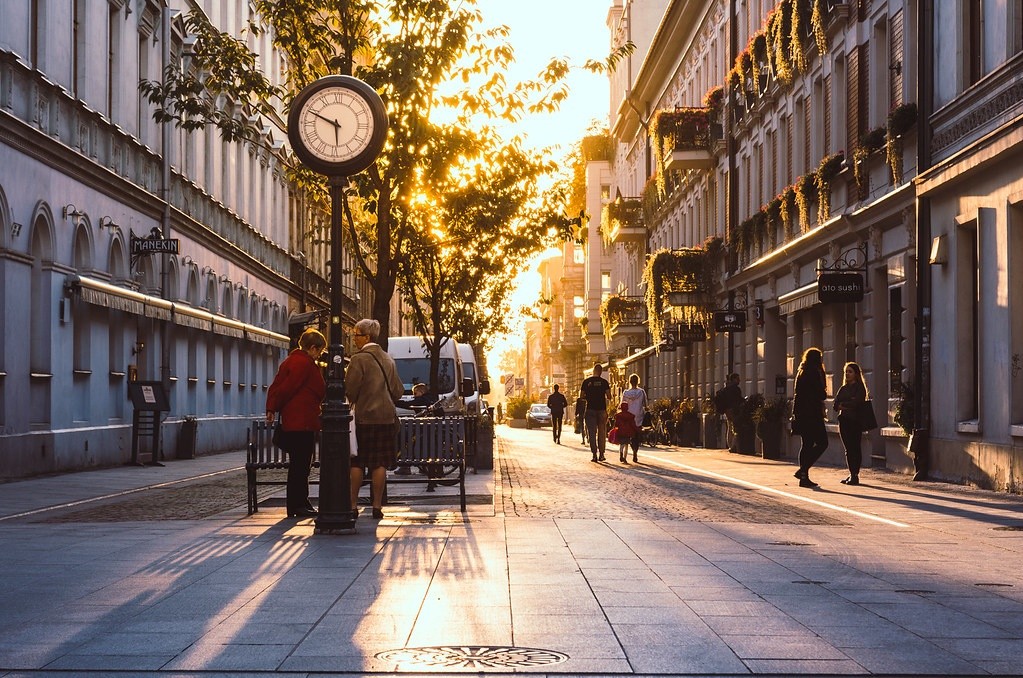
[554,436,556,442]
[581,442,585,445]
[840,472,859,484]
[592,457,596,461]
[794,469,801,479]
[729,447,738,452]
[394,468,411,475]
[800,477,818,488]
[633,453,637,463]
[620,456,627,464]
[599,456,606,461]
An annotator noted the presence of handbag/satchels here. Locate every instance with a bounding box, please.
[609,428,619,444]
[642,412,651,427]
[350,405,357,457]
[842,400,877,431]
[790,419,801,436]
[272,423,289,453]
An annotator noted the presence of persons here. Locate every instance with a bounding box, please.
[497,403,503,425]
[615,402,642,463]
[343,319,405,519]
[394,384,440,486]
[621,374,647,462]
[794,348,830,487]
[832,362,869,485]
[580,364,612,462]
[722,374,744,453]
[575,391,589,445]
[547,384,568,445]
[266,329,328,518]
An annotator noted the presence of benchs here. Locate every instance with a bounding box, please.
[245,414,479,516]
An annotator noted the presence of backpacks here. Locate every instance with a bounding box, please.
[716,385,734,415]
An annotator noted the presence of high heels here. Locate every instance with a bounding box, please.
[352,508,358,519]
[373,508,382,519]
[287,505,319,518]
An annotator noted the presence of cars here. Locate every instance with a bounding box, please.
[526,404,553,431]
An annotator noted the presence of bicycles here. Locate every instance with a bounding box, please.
[394,419,464,475]
[648,410,672,448]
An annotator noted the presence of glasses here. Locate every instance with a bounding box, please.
[353,332,367,337]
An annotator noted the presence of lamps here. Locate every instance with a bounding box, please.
[250,289,257,301]
[259,294,279,311]
[219,274,230,287]
[234,282,248,295]
[182,255,195,271]
[202,266,216,280]
[63,203,82,225]
[100,216,119,235]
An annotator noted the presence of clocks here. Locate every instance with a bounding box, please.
[287,75,390,177]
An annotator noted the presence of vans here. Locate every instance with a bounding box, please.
[388,336,475,435]
[457,342,489,415]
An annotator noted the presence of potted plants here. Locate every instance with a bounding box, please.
[679,392,794,459]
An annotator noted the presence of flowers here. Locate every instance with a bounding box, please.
[703,0,832,127]
[726,103,917,261]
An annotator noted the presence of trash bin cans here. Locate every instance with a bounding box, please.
[179,415,198,459]
